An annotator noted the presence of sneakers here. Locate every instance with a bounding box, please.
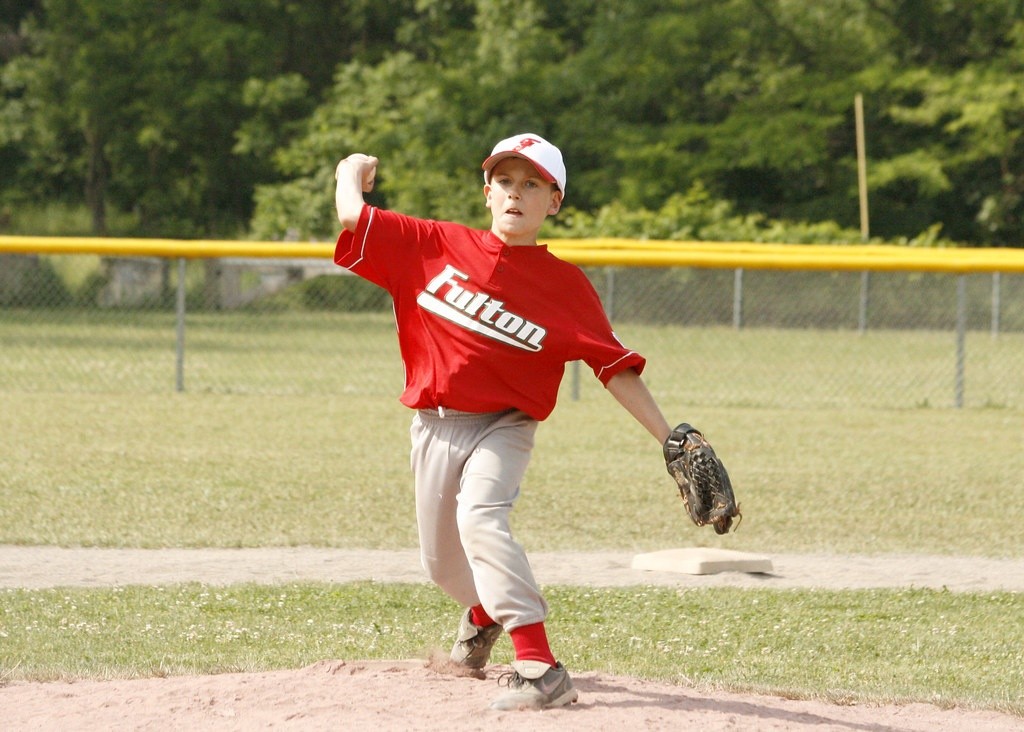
[449,606,502,668]
[491,661,578,710]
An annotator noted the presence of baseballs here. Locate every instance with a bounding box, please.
[347,152,377,184]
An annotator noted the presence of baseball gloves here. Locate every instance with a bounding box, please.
[661,421,744,537]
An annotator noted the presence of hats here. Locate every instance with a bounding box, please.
[483,132,567,199]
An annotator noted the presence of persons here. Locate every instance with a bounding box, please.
[333,133,736,708]
